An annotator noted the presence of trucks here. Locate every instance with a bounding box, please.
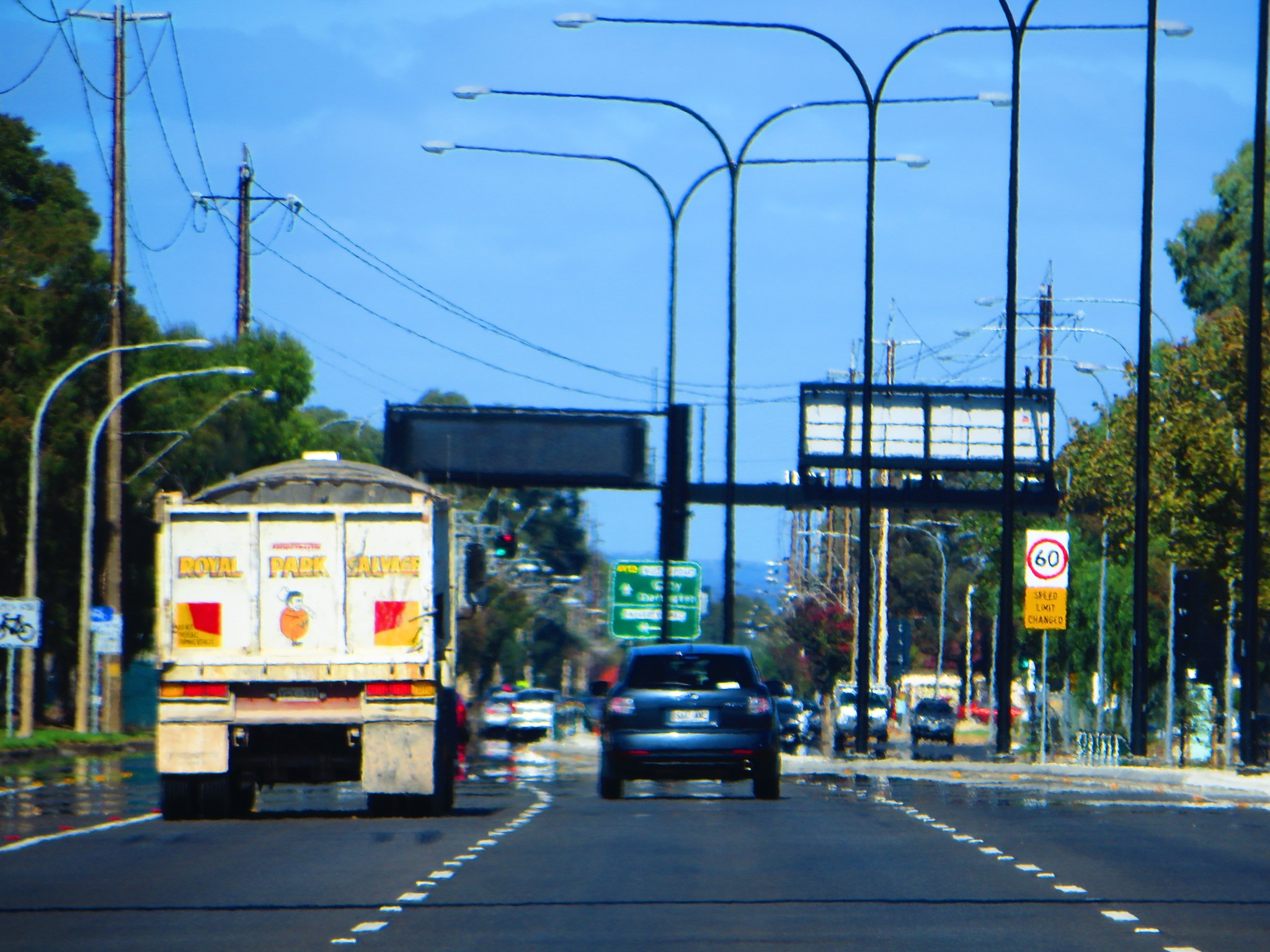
[153,451,488,819]
[828,679,893,760]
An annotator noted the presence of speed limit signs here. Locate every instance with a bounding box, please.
[1028,537,1068,579]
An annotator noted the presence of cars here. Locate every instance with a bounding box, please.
[464,682,607,737]
[909,695,956,742]
[763,679,822,751]
[595,644,786,798]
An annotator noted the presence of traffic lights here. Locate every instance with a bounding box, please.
[1017,641,1035,670]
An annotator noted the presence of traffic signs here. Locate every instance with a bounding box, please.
[606,560,703,638]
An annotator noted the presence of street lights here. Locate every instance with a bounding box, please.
[421,139,930,402]
[976,295,1180,352]
[18,337,279,735]
[450,80,1013,648]
[1085,362,1234,766]
[1071,358,1110,735]
[552,10,1197,754]
[795,528,878,676]
[889,523,946,701]
